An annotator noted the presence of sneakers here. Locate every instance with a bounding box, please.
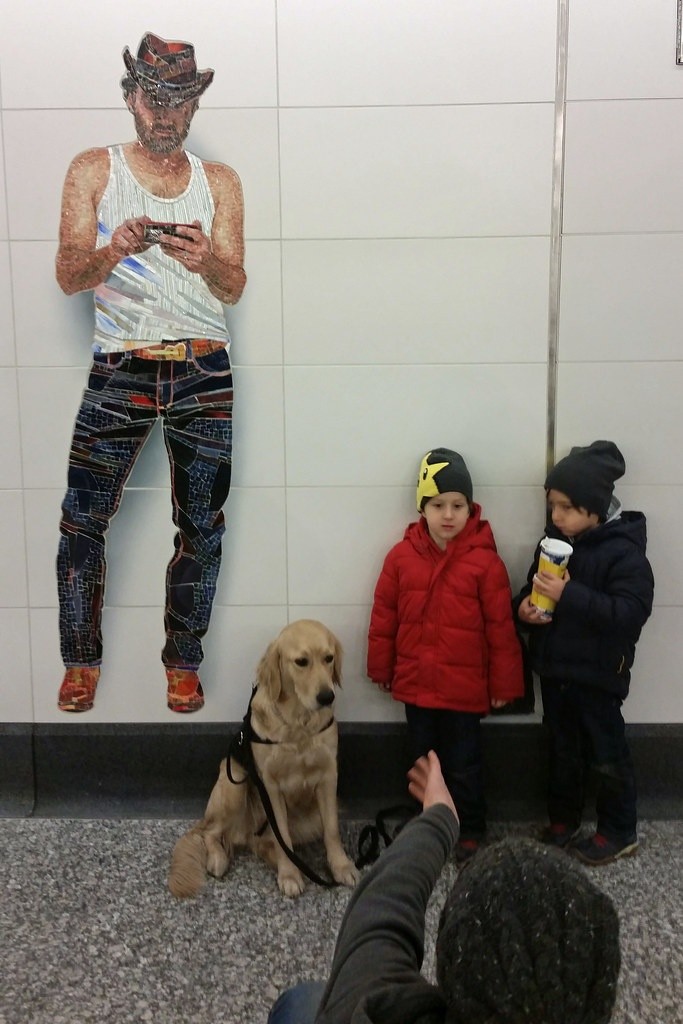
[543,824,582,849]
[453,836,488,867]
[577,833,639,865]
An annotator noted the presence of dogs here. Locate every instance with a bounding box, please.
[167,618,361,898]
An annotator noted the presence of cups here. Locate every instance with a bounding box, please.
[529,573,556,620]
[537,537,573,582]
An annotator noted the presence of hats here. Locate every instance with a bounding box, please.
[544,441,626,514]
[418,447,473,513]
[437,839,620,1024]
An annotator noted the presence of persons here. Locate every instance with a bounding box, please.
[366,447,525,869]
[510,440,655,868]
[267,750,621,1024]
